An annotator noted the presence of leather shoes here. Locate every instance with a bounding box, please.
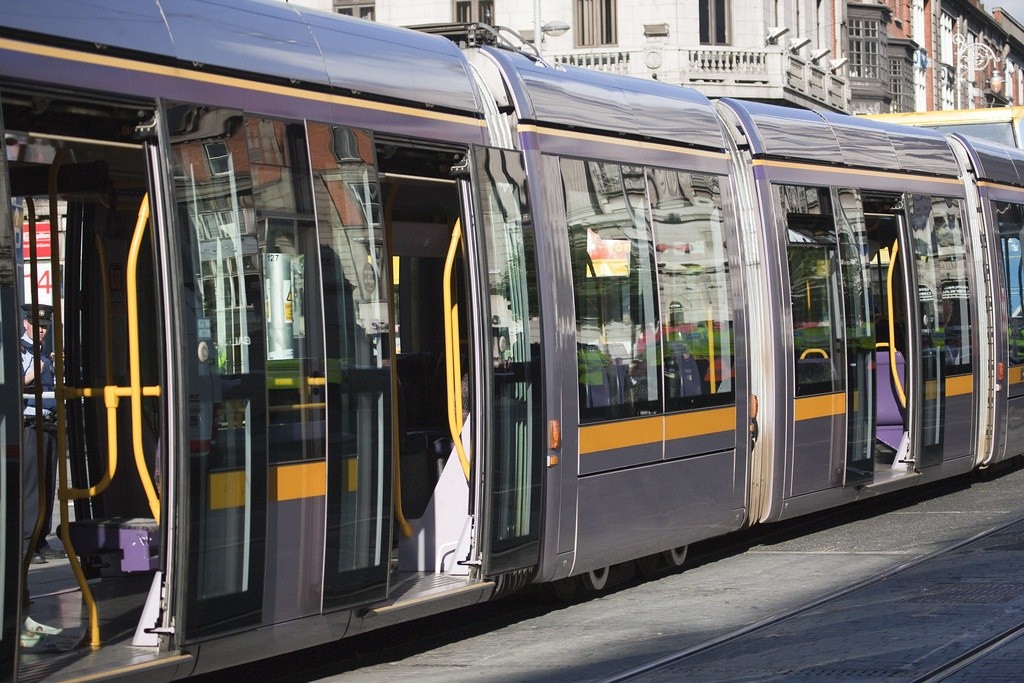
[30,551,45,564]
[39,549,64,559]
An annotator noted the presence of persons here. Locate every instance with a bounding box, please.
[20,304,65,564]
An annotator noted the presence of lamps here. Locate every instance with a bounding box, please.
[788,38,812,53]
[808,49,831,63]
[830,58,851,72]
[766,26,789,45]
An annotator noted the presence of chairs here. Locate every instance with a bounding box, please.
[672,344,700,397]
[872,342,905,425]
[797,347,839,383]
[577,344,609,408]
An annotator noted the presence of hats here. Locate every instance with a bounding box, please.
[20,304,54,326]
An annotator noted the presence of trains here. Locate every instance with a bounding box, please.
[1,1,1024,683]
